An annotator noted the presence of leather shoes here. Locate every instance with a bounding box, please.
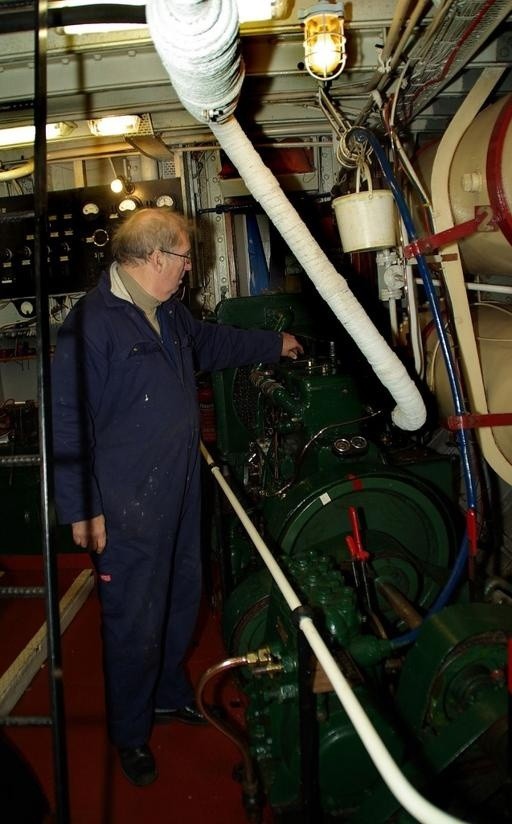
[116,742,160,787]
[152,698,223,726]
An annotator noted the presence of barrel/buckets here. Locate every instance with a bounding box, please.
[331,159,396,253]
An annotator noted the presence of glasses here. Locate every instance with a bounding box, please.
[148,248,193,265]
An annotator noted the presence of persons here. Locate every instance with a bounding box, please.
[52,204,304,787]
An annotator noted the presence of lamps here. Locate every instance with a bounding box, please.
[49,0,294,35]
[88,114,142,137]
[111,176,135,195]
[297,0,348,81]
[0,120,78,146]
